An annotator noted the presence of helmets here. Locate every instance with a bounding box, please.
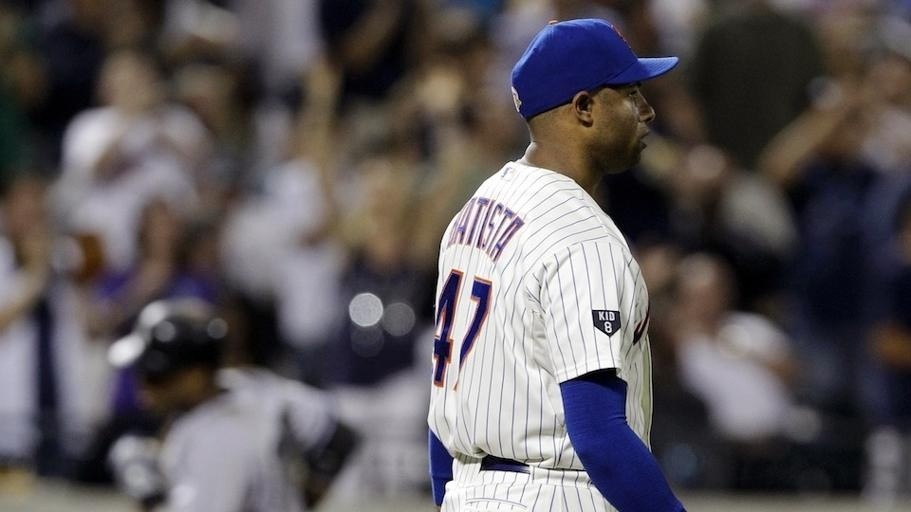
[101,295,237,380]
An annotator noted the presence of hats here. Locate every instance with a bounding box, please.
[510,18,679,120]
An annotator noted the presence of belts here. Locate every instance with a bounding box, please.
[479,455,531,477]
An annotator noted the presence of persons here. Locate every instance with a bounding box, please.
[0,1,911,512]
